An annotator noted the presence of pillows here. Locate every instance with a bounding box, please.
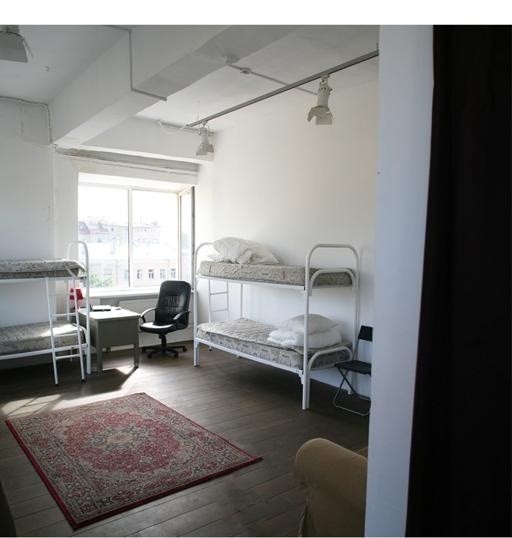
[213,237,279,264]
[280,311,340,334]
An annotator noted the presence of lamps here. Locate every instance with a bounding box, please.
[194,77,337,163]
[67,288,83,308]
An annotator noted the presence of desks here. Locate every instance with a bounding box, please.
[72,303,143,373]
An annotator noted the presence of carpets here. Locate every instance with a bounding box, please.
[4,390,264,532]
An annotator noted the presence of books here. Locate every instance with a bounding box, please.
[93,304,111,311]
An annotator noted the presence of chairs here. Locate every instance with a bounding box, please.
[332,326,372,418]
[137,278,191,360]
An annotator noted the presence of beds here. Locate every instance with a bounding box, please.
[187,237,361,412]
[0,236,92,387]
